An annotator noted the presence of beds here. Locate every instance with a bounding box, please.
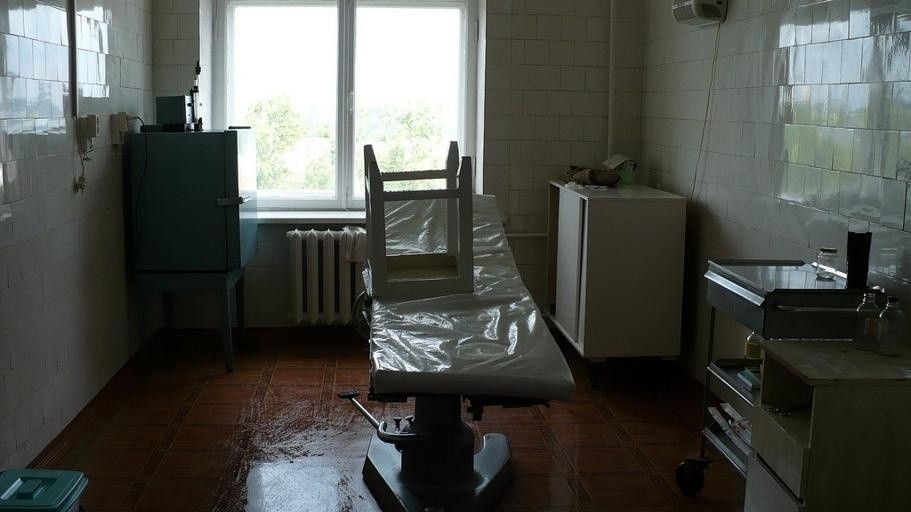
[337,193,576,511]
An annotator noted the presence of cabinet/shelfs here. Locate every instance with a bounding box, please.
[546,185,689,364]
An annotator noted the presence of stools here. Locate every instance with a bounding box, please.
[364,141,475,298]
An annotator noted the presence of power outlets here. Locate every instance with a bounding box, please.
[77,111,129,139]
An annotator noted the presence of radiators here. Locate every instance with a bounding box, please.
[285,225,367,324]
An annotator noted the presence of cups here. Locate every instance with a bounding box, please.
[619,159,636,186]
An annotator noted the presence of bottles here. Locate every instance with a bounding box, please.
[744,331,765,371]
[851,292,882,352]
[815,247,839,280]
[874,295,908,357]
